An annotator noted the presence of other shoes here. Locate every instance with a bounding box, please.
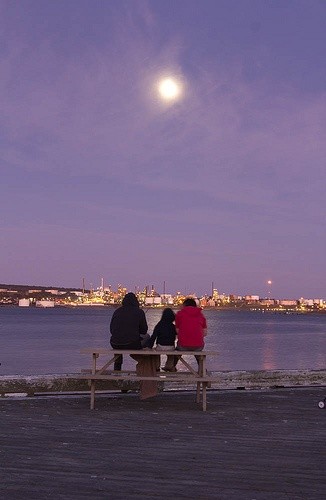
[162,367,172,372]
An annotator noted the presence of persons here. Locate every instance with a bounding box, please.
[150,308,177,370]
[162,297,207,372]
[109,292,150,371]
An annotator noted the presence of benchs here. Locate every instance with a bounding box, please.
[78,369,220,383]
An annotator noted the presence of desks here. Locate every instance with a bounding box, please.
[80,348,217,410]
